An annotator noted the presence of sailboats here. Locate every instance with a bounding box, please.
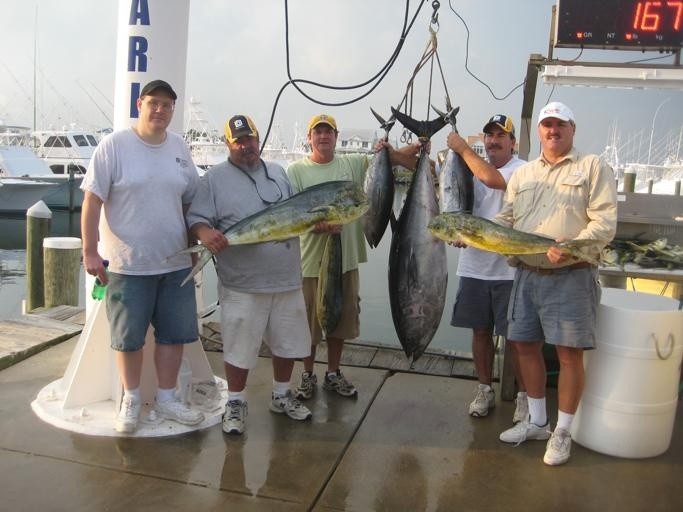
[593,92,682,196]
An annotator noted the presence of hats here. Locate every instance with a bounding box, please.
[483,114,515,139]
[141,80,177,104]
[225,114,258,144]
[308,115,338,131]
[537,102,575,126]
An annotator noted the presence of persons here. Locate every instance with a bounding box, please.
[375,111,530,424]
[446,100,617,469]
[285,114,431,401]
[80,80,208,432]
[186,113,331,437]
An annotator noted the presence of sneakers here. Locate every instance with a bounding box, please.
[114,369,358,434]
[470,383,572,465]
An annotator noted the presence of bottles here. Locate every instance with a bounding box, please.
[90,260,109,301]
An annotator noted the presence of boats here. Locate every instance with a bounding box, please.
[0,142,89,212]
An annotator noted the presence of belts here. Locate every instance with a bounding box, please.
[518,261,598,275]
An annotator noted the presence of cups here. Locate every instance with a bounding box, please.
[623,166,637,193]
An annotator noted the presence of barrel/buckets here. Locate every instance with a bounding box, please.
[569,286,683,459]
